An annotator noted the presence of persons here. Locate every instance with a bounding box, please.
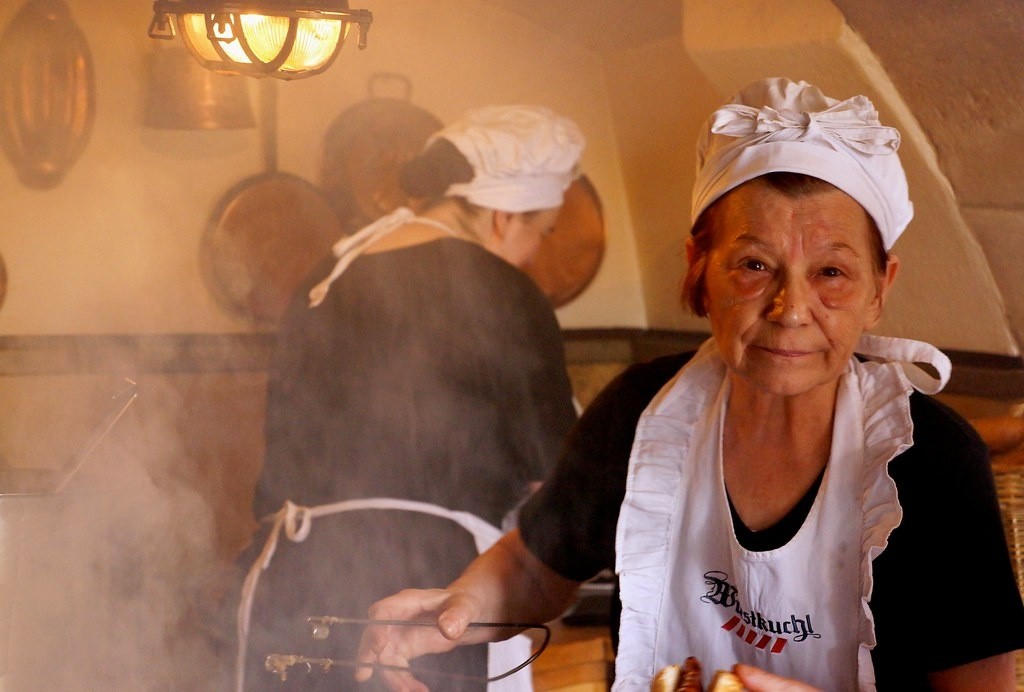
[233,101,586,692]
[357,76,1024,691]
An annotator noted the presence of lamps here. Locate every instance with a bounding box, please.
[147,0,373,82]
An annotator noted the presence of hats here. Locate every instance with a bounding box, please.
[689,76,913,251]
[422,102,587,213]
